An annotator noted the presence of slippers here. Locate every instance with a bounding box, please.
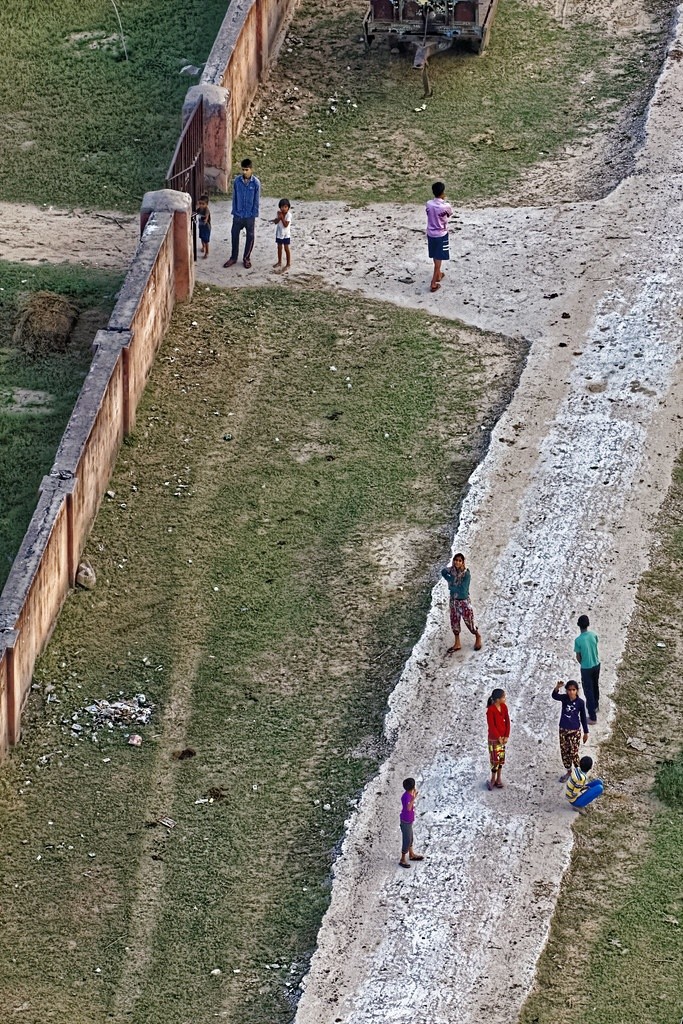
[447,646,461,653]
[430,283,441,292]
[436,272,445,282]
[474,644,482,651]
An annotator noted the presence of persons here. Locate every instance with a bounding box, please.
[566,756,604,815]
[399,778,424,868]
[196,196,211,258]
[574,615,601,724]
[223,159,260,268]
[552,680,589,782]
[442,553,482,652]
[486,689,511,789]
[273,199,292,271]
[426,182,453,292]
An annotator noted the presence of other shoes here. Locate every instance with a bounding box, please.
[495,781,504,788]
[410,855,424,861]
[559,774,569,783]
[585,717,598,725]
[486,780,493,791]
[398,862,411,868]
[223,257,238,267]
[242,256,252,268]
[572,805,588,815]
